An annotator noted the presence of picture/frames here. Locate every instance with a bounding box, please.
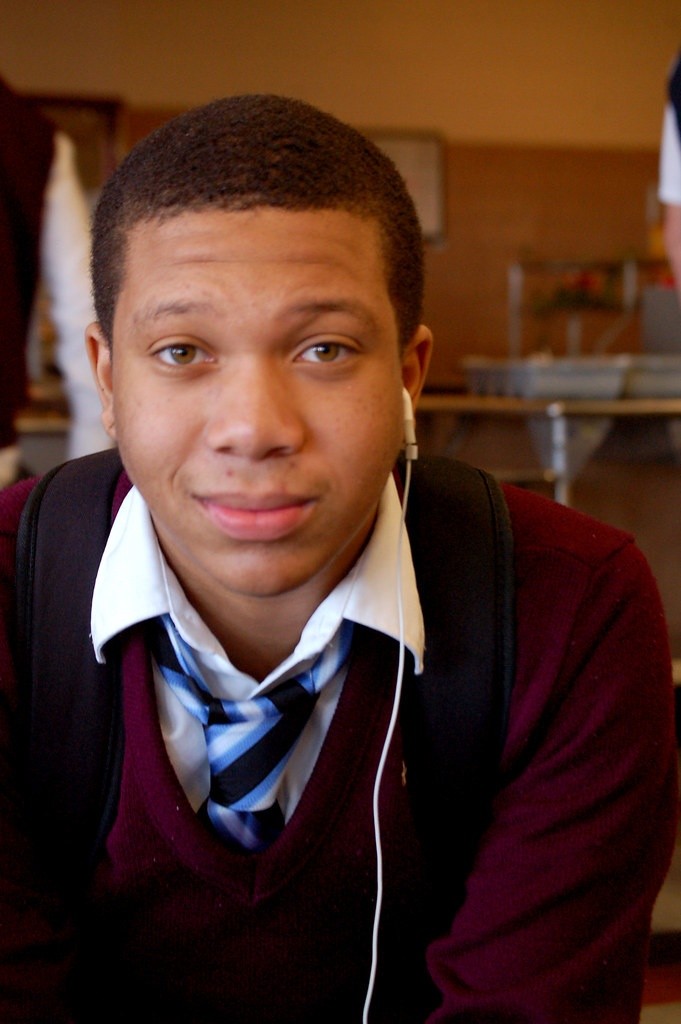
[358,131,447,240]
[26,95,123,407]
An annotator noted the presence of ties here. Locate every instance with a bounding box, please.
[144,608,346,866]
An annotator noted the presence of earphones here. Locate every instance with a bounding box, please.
[401,389,418,462]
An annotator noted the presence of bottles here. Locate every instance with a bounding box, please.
[555,265,610,307]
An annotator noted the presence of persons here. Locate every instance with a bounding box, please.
[0,76,116,486]
[656,50,681,294]
[0,94,681,1024]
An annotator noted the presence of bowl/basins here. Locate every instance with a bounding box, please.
[452,356,627,397]
[623,355,680,397]
